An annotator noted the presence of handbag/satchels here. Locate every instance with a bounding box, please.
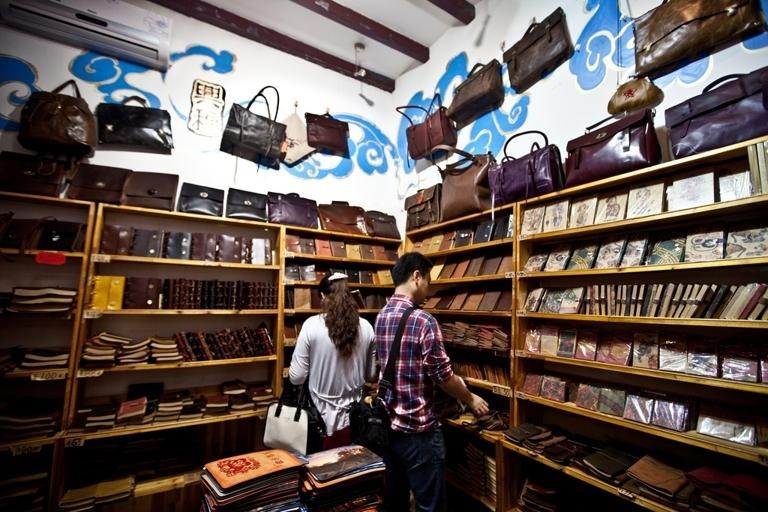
[304,111,350,158]
[95,96,175,155]
[397,1,767,511]
[352,396,390,442]
[219,85,286,170]
[17,79,96,157]
[262,401,325,453]
[2,154,401,511]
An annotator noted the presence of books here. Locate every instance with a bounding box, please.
[578,281,768,322]
[447,440,499,505]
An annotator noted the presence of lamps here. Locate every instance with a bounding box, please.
[353,42,367,77]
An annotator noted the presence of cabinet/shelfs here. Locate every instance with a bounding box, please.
[443,438,502,512]
[0,192,95,512]
[57,202,282,495]
[500,134,768,512]
[405,203,517,437]
[283,227,403,373]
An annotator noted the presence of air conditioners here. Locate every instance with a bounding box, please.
[0,0,171,75]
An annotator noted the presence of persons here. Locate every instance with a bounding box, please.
[374,251,490,510]
[288,269,380,452]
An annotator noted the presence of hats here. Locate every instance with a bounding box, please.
[318,272,345,292]
